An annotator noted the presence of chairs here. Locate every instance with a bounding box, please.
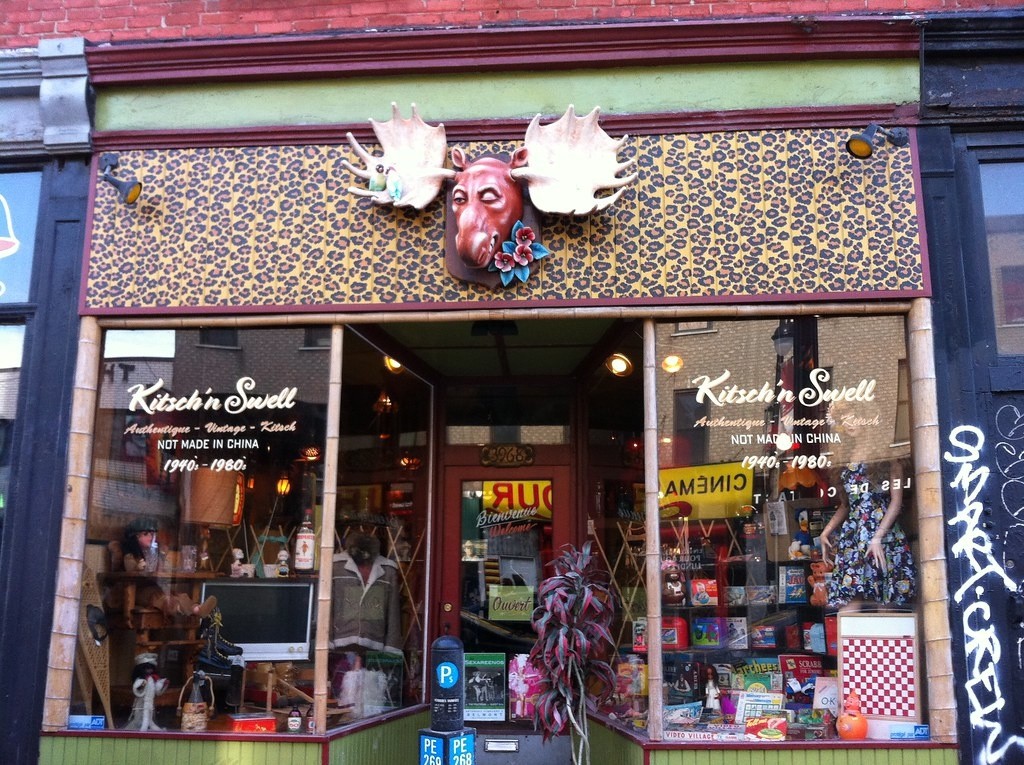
[96,539,227,709]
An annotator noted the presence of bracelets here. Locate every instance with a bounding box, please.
[878,527,886,533]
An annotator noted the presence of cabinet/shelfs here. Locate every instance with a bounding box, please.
[618,544,835,664]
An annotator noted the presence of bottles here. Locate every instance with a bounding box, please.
[295,509,315,572]
[286,704,315,735]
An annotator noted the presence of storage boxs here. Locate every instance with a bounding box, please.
[583,565,838,742]
[226,712,279,734]
[67,715,105,730]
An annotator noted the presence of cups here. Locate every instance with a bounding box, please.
[181,545,197,573]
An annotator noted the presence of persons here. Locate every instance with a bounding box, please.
[330,528,402,718]
[396,540,412,561]
[658,559,687,607]
[121,516,217,630]
[694,581,706,605]
[702,665,722,713]
[608,676,640,718]
[120,651,171,732]
[673,673,691,692]
[470,668,504,704]
[462,540,486,561]
[819,391,916,613]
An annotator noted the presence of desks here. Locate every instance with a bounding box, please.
[96,571,226,630]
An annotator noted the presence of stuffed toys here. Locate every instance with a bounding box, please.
[789,509,814,558]
[808,563,834,606]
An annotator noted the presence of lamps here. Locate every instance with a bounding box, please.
[180,467,238,570]
[276,475,291,495]
[606,352,633,377]
[662,355,683,372]
[400,455,422,470]
[373,393,399,413]
[302,447,322,460]
[383,355,404,374]
[99,152,143,205]
[246,474,255,489]
[843,123,911,160]
[771,318,795,356]
